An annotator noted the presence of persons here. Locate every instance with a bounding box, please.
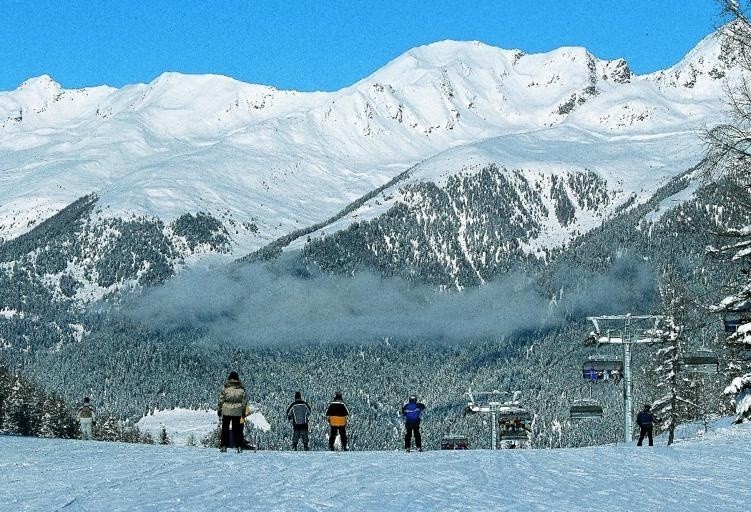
[498,417,532,433]
[75,398,97,441]
[326,392,349,451]
[402,396,426,453]
[287,392,311,450]
[217,371,249,453]
[441,442,468,450]
[591,366,624,385]
[637,404,662,446]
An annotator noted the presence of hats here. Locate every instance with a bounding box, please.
[295,392,301,399]
[334,393,343,401]
[228,371,238,380]
[409,395,417,402]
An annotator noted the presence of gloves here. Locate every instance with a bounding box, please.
[218,411,221,416]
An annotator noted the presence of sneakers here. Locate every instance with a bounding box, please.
[237,446,242,453]
[220,446,227,452]
